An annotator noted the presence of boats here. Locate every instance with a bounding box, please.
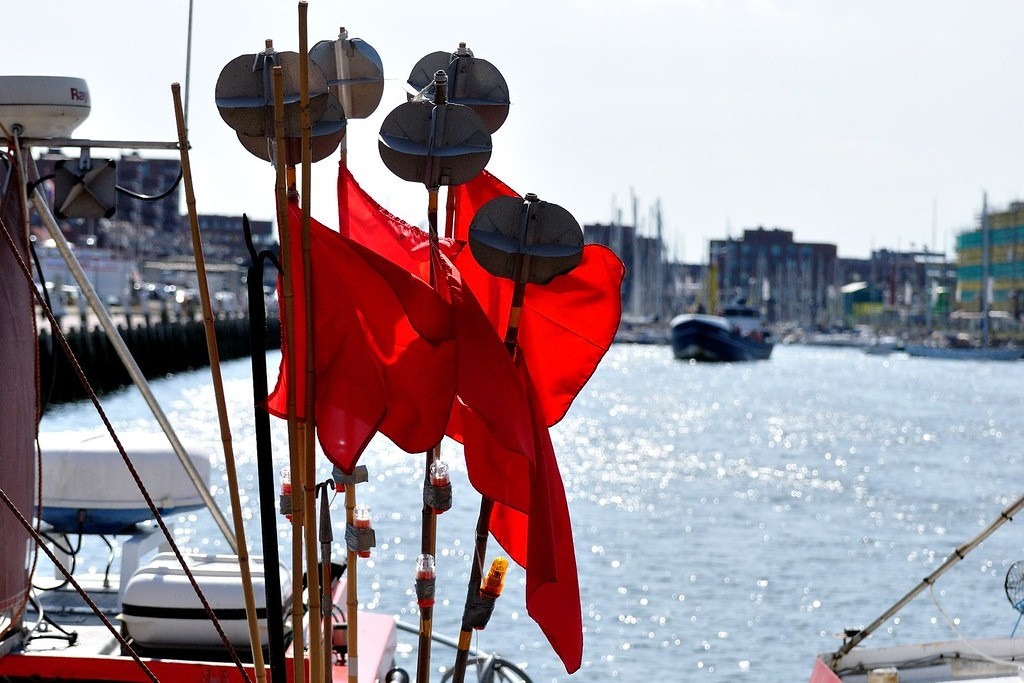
[809,495,1024,683]
[907,344,1024,361]
[670,255,777,362]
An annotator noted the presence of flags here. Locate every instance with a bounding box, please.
[266,196,464,471]
[452,170,621,431]
[338,159,582,672]
